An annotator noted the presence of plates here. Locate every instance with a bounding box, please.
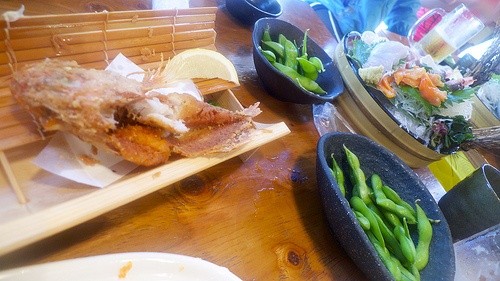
[443,150,475,180]
[0,252,242,281]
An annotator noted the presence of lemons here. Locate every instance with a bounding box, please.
[161,48,239,84]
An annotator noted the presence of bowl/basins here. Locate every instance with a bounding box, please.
[315,130,456,281]
[252,17,345,105]
[226,0,283,25]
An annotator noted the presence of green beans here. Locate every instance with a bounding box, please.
[257,26,328,97]
[327,143,441,281]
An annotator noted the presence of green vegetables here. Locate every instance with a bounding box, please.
[348,38,482,112]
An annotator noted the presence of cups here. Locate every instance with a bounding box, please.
[408,3,486,65]
[438,163,500,244]
